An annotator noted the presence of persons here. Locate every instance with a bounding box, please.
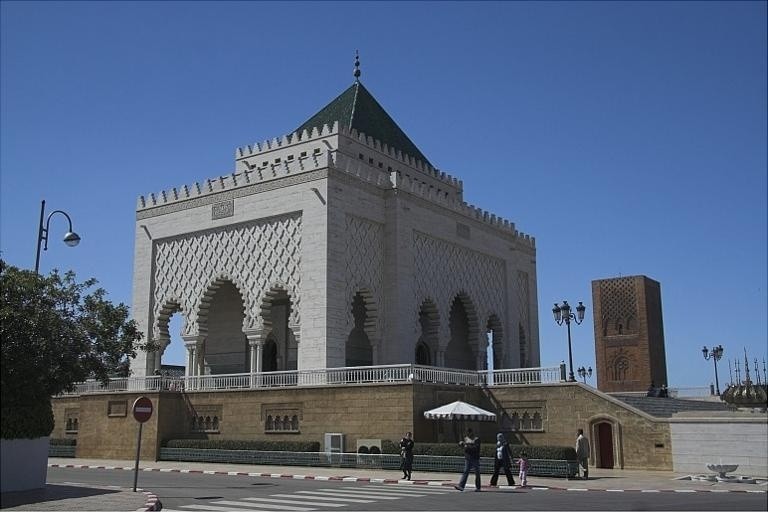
[514,451,529,487]
[488,432,517,486]
[659,384,668,397]
[152,368,163,388]
[162,369,174,390]
[575,427,591,479]
[400,431,414,480]
[452,427,482,492]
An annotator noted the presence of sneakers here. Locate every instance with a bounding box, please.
[454,480,527,492]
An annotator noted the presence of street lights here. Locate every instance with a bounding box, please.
[552,301,586,382]
[577,366,592,383]
[702,345,724,396]
[34,200,80,274]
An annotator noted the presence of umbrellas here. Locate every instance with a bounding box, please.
[423,397,497,424]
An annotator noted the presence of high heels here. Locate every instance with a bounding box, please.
[401,474,411,481]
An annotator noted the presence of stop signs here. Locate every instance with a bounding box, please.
[131,396,153,423]
